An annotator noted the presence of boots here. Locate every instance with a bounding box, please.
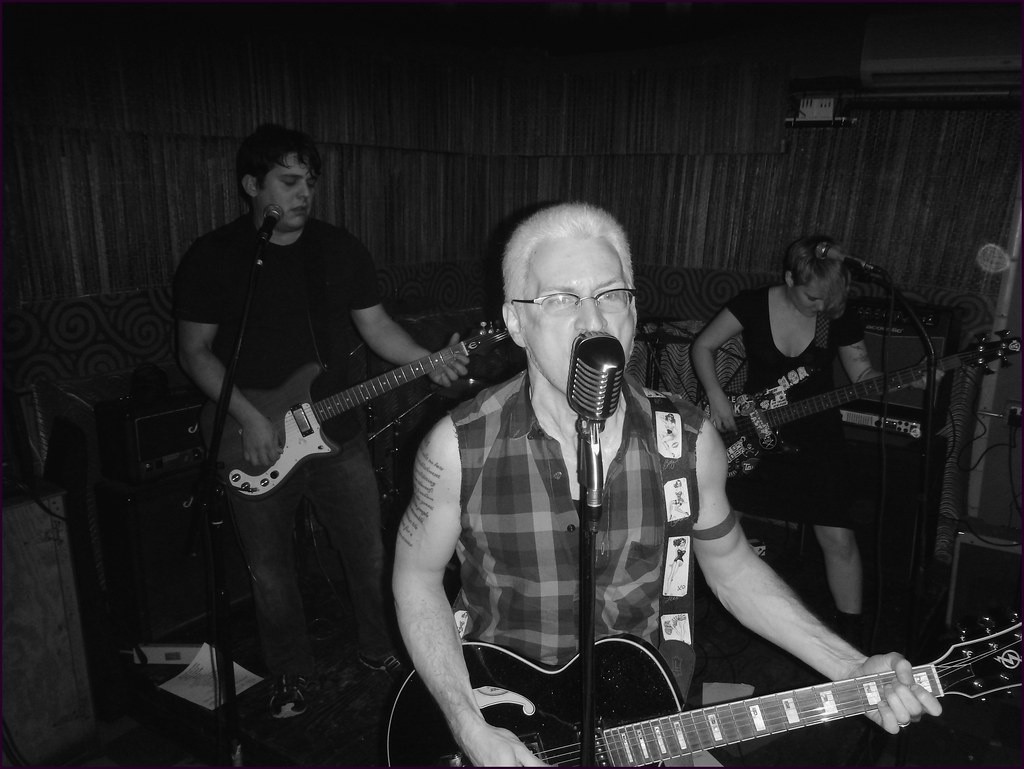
[836,612,864,650]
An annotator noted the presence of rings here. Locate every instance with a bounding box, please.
[899,721,911,728]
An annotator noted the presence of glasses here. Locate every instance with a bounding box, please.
[508,286,639,314]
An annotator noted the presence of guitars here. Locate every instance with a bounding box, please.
[381,608,1023,769]
[201,319,512,504]
[700,329,1023,484]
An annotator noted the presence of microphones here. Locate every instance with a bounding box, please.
[565,331,625,536]
[256,205,284,244]
[814,242,882,275]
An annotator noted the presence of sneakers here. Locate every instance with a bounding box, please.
[347,652,403,674]
[269,663,309,718]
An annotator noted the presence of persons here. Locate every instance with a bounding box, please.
[392,201,941,769]
[691,236,944,643]
[171,123,470,719]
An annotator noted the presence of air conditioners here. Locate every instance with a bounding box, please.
[858,8,1024,98]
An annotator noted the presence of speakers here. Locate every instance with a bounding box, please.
[830,297,958,438]
[947,520,1024,642]
[92,463,252,648]
[795,419,928,597]
